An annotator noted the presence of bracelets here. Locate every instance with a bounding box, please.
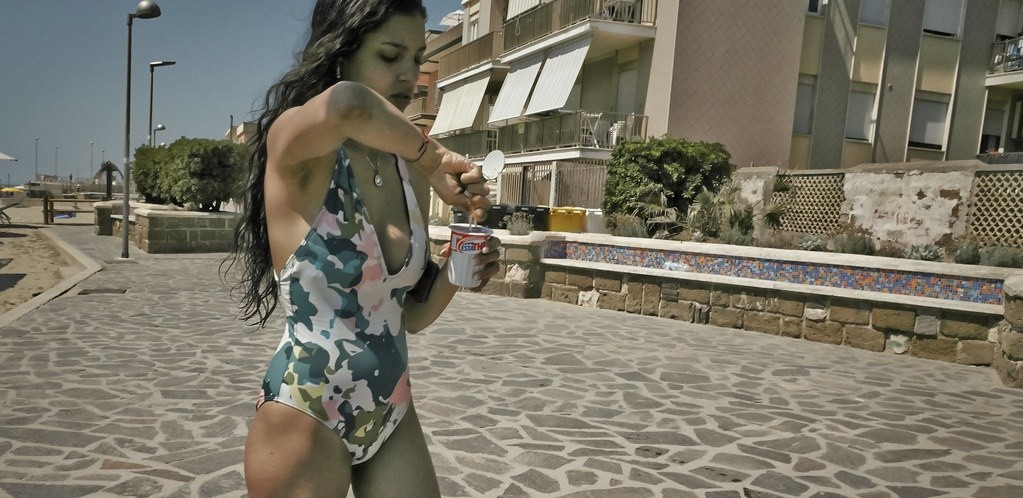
[399,130,430,162]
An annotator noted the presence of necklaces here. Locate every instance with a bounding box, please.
[354,142,384,187]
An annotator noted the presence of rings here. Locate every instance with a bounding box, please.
[466,200,472,209]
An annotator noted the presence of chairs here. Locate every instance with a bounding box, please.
[573,111,639,149]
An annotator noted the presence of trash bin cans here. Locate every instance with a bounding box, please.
[547,207,586,233]
[584,209,605,233]
[451,204,469,223]
[477,203,512,229]
[512,205,551,231]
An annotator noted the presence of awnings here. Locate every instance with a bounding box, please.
[486,49,545,124]
[524,35,594,117]
[427,70,495,136]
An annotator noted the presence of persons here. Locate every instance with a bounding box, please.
[216,0,501,498]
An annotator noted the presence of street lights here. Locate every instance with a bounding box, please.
[36,136,40,173]
[154,124,166,149]
[148,59,177,143]
[55,146,59,175]
[102,150,104,163]
[121,0,162,258]
[90,142,94,178]
